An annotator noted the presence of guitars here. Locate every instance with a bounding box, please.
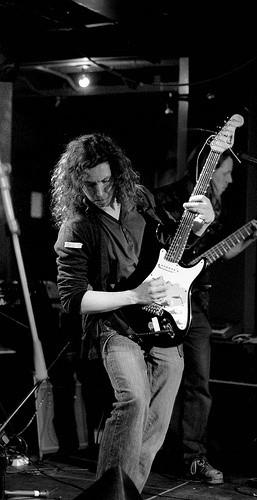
[109,113,245,348]
[187,222,253,270]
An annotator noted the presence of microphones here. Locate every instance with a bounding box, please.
[232,150,257,164]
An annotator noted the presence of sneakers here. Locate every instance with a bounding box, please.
[176,456,224,484]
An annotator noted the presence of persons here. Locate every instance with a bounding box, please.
[148,139,257,485]
[52,134,217,491]
